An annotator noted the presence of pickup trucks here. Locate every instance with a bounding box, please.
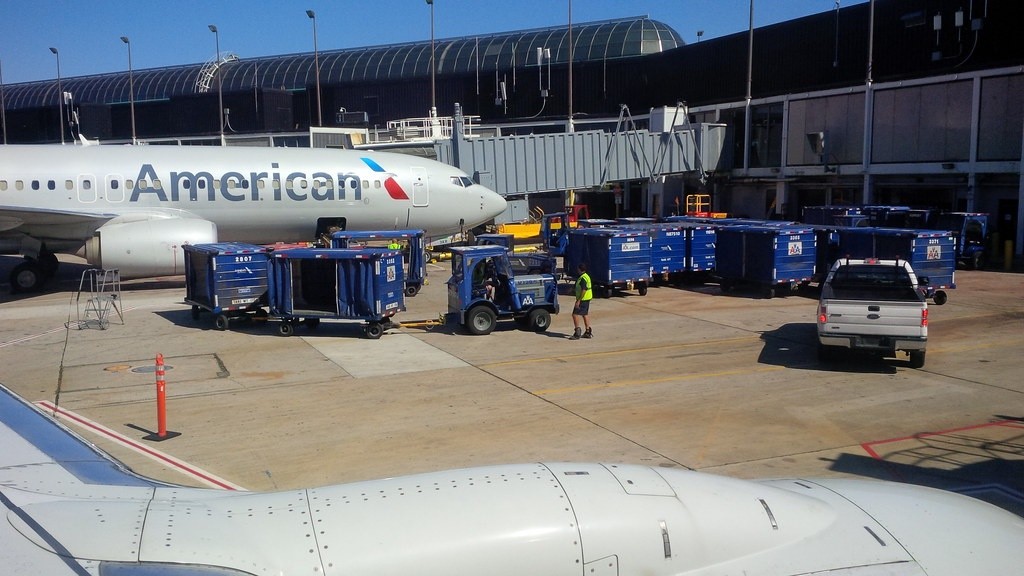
[816,257,928,368]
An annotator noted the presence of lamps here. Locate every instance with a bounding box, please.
[771,168,779,173]
[942,164,956,170]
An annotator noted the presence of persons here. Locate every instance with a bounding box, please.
[572,263,594,340]
[468,259,501,303]
[388,238,400,250]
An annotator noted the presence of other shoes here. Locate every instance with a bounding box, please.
[580,327,593,338]
[568,327,581,340]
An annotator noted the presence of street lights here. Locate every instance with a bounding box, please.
[208,25,224,135]
[426,0,436,107]
[306,10,322,127]
[50,47,64,144]
[120,37,137,145]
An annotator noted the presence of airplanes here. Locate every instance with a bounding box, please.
[0,133,507,295]
[0,384,1024,576]
